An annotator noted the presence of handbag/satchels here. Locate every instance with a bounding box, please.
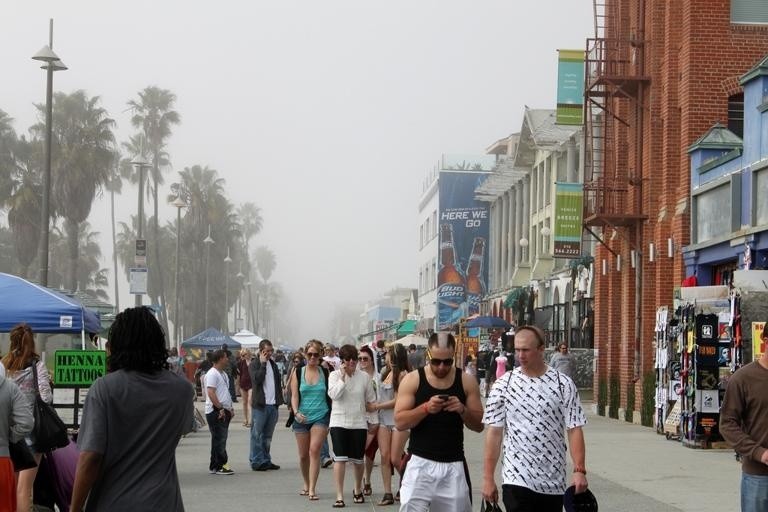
[9,438,37,472]
[30,359,70,452]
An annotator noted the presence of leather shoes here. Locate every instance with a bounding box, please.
[251,464,268,471]
[268,464,280,469]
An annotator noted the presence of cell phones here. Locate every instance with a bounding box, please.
[259,350,267,357]
[340,359,344,364]
[439,395,449,401]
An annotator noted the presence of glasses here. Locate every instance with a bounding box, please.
[428,352,454,365]
[345,357,356,361]
[358,357,368,361]
[306,353,319,357]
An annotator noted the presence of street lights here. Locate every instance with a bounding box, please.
[247,279,274,346]
[236,261,245,332]
[223,243,233,333]
[202,225,216,330]
[32,17,69,290]
[172,184,184,348]
[130,132,151,311]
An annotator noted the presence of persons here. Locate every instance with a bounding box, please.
[718,346,731,367]
[391,333,484,510]
[287,338,425,507]
[718,322,768,512]
[480,325,589,511]
[169,340,307,476]
[483,349,515,396]
[462,349,485,386]
[672,369,679,379]
[673,383,680,396]
[0,360,35,512]
[67,306,196,511]
[550,343,573,381]
[0,323,54,511]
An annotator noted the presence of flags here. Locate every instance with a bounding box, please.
[554,49,584,125]
[552,182,585,258]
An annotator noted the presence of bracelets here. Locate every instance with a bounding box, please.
[422,404,430,415]
[573,466,586,475]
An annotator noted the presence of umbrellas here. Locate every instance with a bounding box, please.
[464,315,513,341]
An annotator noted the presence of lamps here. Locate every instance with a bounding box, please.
[518,237,529,263]
[541,225,552,254]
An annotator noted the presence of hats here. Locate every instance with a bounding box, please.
[563,486,598,511]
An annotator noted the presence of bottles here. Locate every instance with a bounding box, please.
[438,221,466,332]
[465,235,486,320]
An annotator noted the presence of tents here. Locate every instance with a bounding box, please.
[177,326,264,355]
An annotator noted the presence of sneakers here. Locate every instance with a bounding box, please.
[321,458,332,468]
[215,464,235,475]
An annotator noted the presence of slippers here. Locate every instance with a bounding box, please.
[299,478,400,508]
